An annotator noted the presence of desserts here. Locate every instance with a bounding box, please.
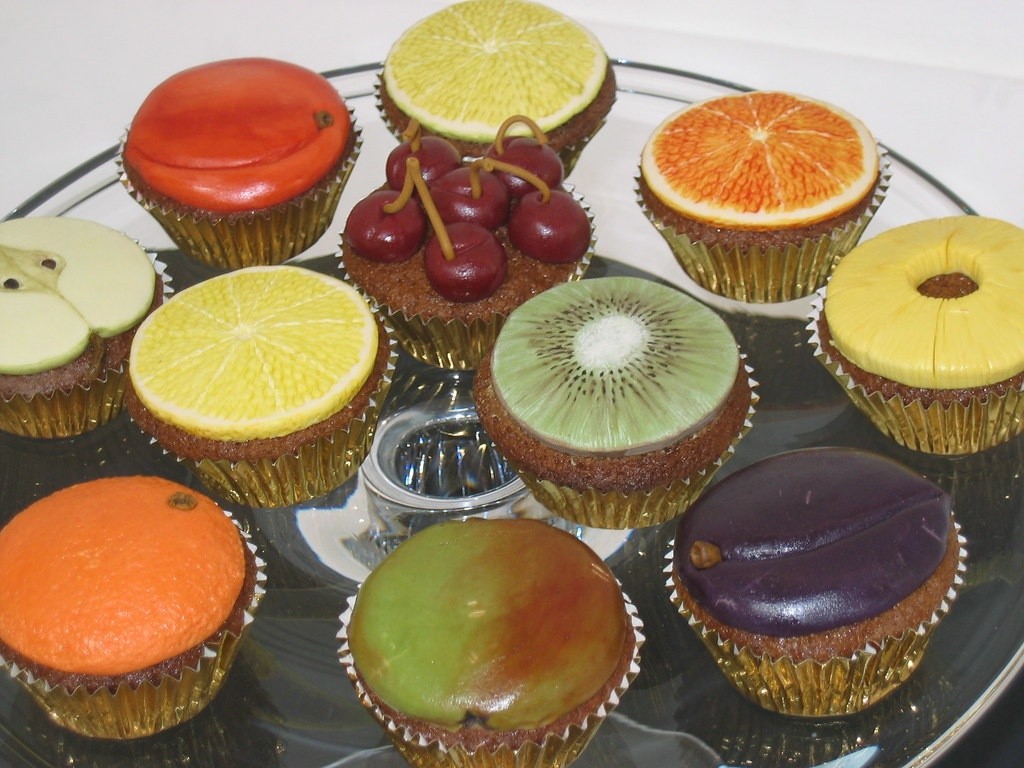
[0,0,1024,768]
